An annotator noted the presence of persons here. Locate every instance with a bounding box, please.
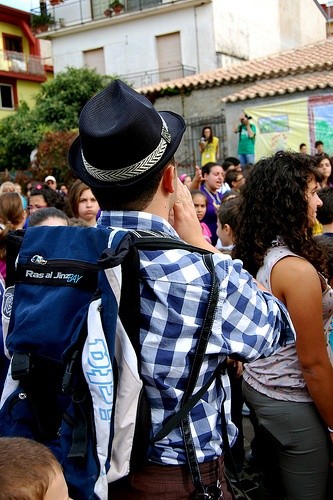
[0,111,332,500]
[0,80,296,500]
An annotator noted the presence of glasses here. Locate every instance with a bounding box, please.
[27,206,48,212]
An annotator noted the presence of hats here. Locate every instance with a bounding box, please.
[66,78,187,188]
[45,175,56,183]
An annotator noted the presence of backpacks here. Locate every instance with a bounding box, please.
[0,225,223,500]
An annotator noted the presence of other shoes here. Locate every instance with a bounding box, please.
[225,466,243,480]
[242,402,251,417]
[242,449,257,467]
